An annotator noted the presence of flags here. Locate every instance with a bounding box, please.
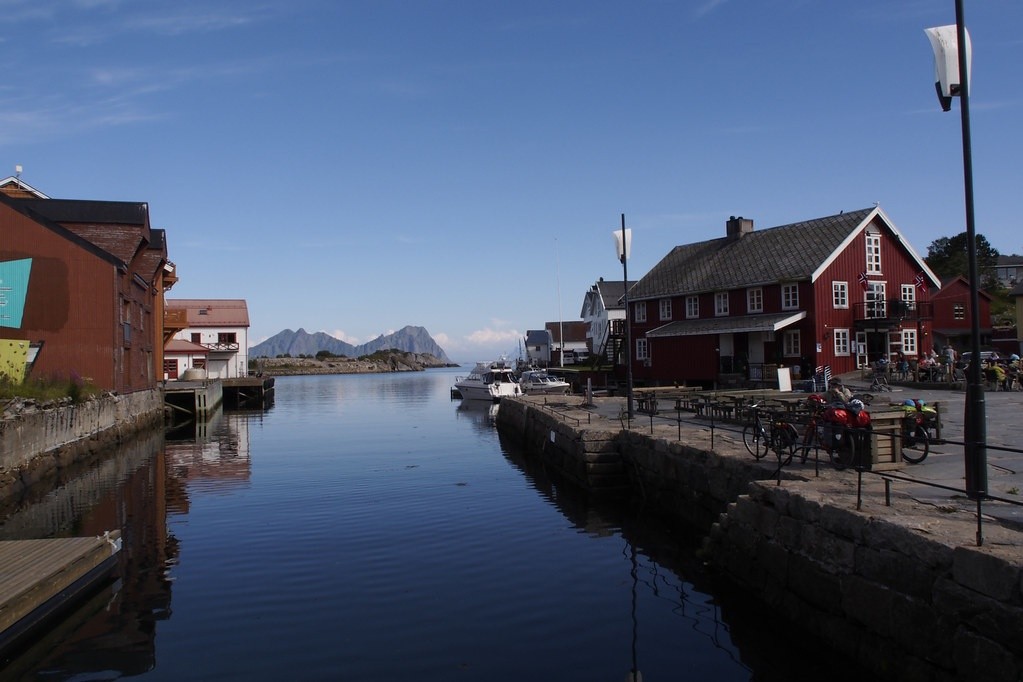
[913,272,926,294]
[857,270,869,291]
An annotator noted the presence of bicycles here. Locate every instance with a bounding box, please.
[798,399,856,471]
[901,421,930,464]
[743,398,795,466]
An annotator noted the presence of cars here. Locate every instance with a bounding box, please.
[960,352,1001,361]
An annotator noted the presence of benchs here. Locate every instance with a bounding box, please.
[633,397,811,424]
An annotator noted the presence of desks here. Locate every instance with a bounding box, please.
[694,388,826,425]
[632,386,703,415]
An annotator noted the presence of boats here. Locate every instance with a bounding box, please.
[516,360,571,396]
[453,354,528,403]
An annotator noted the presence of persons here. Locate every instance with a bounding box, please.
[876,345,1023,391]
[800,354,812,381]
[809,377,852,404]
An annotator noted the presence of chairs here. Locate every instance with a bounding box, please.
[870,359,1010,392]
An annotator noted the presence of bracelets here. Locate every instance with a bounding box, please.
[835,391,839,393]
